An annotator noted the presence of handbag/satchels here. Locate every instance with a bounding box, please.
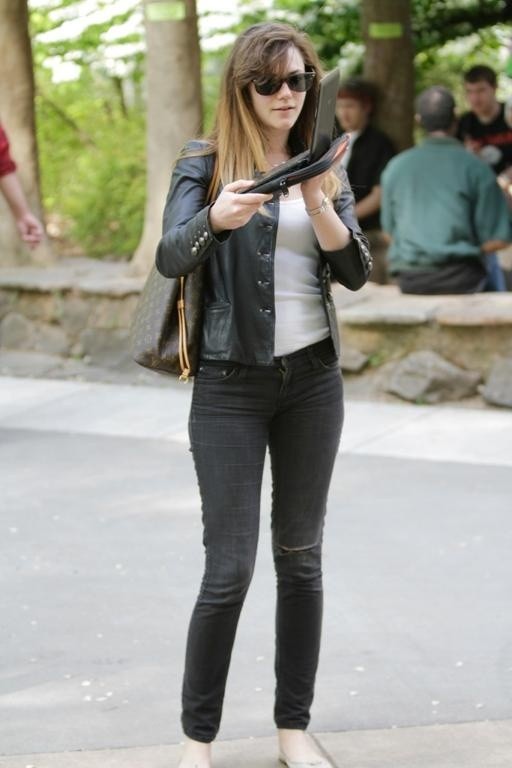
[244,135,351,198]
[125,241,214,386]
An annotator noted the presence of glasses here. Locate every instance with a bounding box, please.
[249,66,316,96]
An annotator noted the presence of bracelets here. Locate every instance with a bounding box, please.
[304,195,331,218]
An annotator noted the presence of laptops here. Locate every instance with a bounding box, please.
[237,66,341,192]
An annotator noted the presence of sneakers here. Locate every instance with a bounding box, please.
[174,740,215,768]
[278,738,335,768]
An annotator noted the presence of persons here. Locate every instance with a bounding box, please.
[334,78,397,286]
[0,118,44,251]
[150,23,376,768]
[456,62,512,176]
[379,84,511,298]
